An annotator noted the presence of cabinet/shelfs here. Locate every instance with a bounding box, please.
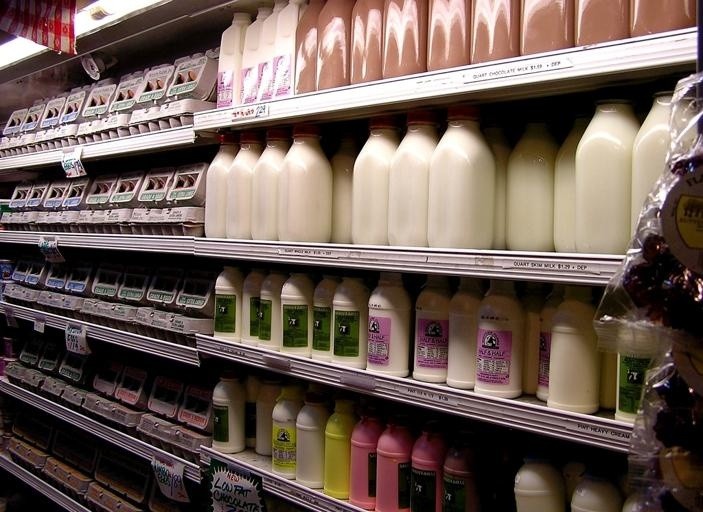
[2,3,202,511]
[194,25,700,510]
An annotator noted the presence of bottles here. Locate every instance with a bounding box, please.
[204,1,701,111]
[215,264,658,425]
[210,370,651,511]
[204,75,703,256]
